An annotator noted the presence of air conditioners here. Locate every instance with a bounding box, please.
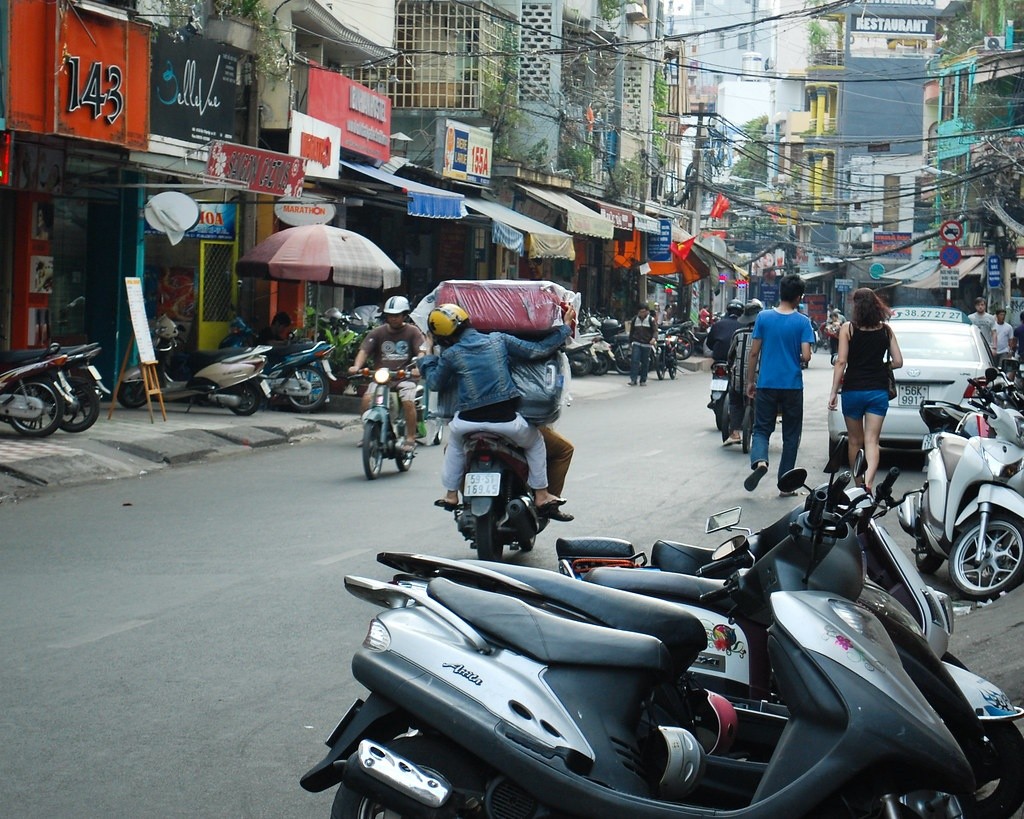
[982,34,1006,53]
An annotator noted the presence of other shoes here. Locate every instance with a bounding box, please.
[544,509,574,522]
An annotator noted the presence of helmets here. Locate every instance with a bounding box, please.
[383,295,411,314]
[427,303,468,336]
[726,299,745,314]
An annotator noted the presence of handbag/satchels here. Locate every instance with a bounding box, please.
[884,325,897,400]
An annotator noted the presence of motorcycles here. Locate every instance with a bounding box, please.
[294,369,1023,819]
[564,307,725,381]
[0,294,432,480]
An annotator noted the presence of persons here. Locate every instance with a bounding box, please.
[720,298,764,447]
[968,297,997,358]
[826,289,903,498]
[531,421,575,522]
[257,312,291,368]
[627,303,658,387]
[829,306,847,325]
[742,274,816,496]
[415,298,575,511]
[705,299,744,362]
[993,309,1015,368]
[827,314,844,355]
[1011,312,1024,365]
[349,296,425,452]
[652,300,717,328]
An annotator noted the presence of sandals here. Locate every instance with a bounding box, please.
[744,465,767,491]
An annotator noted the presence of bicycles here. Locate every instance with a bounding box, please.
[810,319,820,353]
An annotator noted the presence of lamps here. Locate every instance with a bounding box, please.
[133,12,203,44]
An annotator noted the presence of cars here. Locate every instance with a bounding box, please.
[827,306,1021,467]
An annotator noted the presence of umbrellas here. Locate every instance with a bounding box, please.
[235,223,404,414]
[611,222,732,288]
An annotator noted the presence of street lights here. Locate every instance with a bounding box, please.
[729,176,777,201]
[920,165,982,198]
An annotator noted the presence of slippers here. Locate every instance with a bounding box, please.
[779,490,798,497]
[723,436,741,446]
[401,439,416,452]
[536,497,567,510]
[434,499,457,507]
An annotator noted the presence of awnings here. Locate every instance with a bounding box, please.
[344,160,661,261]
[879,253,985,289]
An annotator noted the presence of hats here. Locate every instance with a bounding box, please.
[737,297,764,324]
